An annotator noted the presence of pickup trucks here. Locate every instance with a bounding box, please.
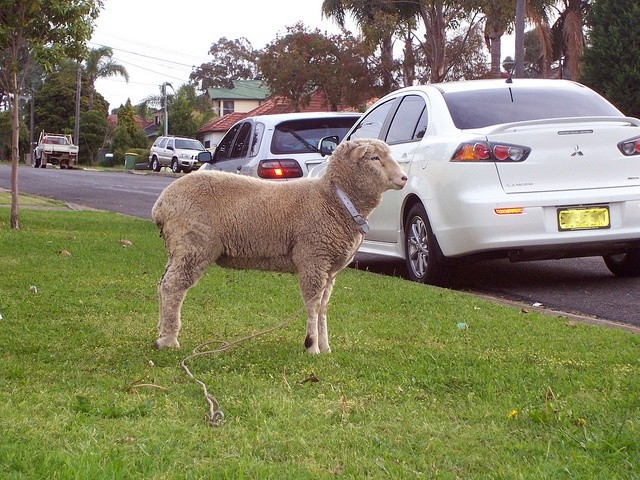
[34,129,79,169]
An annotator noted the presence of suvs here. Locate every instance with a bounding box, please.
[149,134,212,172]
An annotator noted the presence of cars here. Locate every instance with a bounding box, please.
[304,78,640,286]
[197,110,364,183]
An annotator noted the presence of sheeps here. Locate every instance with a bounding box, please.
[151,137,409,357]
